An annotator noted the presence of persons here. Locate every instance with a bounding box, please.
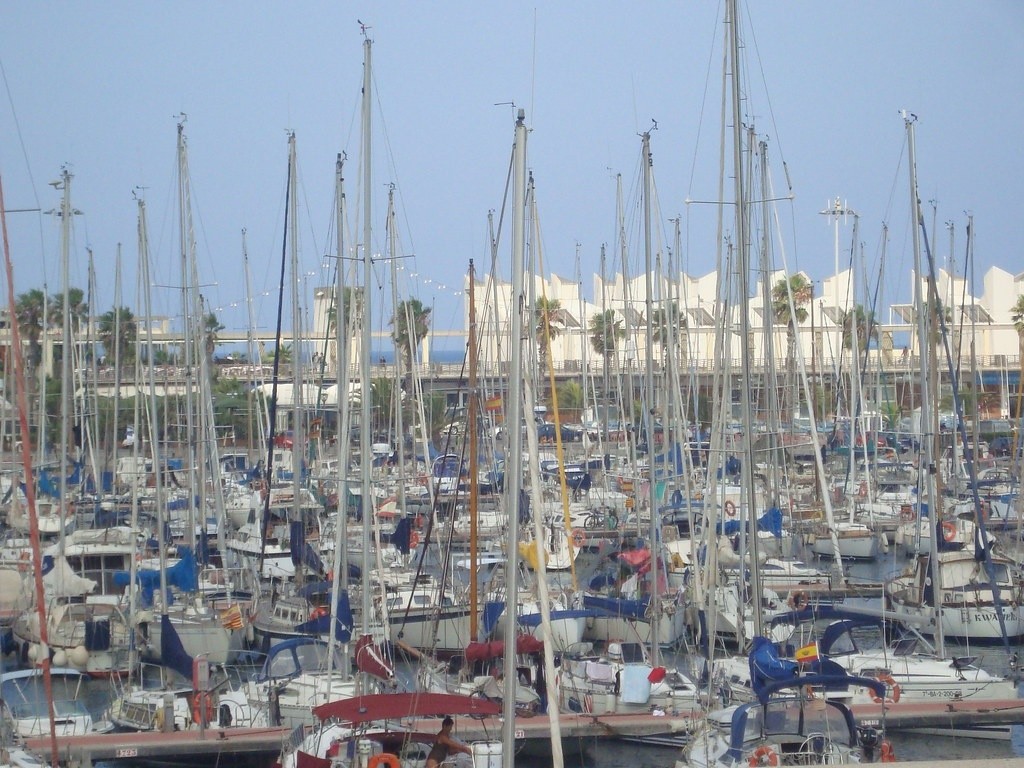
[380,356,386,371]
[253,460,267,478]
[901,346,909,366]
[312,351,325,373]
[425,718,472,768]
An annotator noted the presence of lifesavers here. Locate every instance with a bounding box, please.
[859,484,867,496]
[409,531,418,548]
[193,692,211,724]
[572,530,585,546]
[943,523,956,541]
[312,609,325,619]
[725,501,737,516]
[901,508,915,521]
[751,745,778,767]
[870,677,900,702]
[881,742,895,762]
[368,753,402,768]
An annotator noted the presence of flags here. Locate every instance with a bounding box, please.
[310,416,322,441]
[219,603,246,631]
[485,395,502,411]
[795,643,822,661]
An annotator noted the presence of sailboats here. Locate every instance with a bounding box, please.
[0,3,1023,767]
